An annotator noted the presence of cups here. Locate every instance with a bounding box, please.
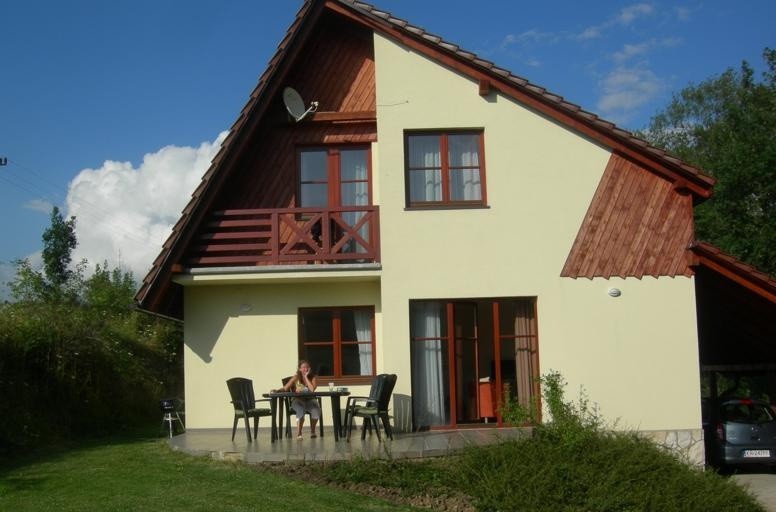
[329,383,333,391]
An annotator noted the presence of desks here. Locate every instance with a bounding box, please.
[262,389,350,442]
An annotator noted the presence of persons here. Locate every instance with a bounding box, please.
[270,359,321,440]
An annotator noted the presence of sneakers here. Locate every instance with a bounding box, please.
[296,433,303,441]
[309,433,317,439]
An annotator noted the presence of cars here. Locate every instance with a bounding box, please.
[712,395,775,476]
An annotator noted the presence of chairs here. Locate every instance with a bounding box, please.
[225,375,324,444]
[343,372,397,444]
